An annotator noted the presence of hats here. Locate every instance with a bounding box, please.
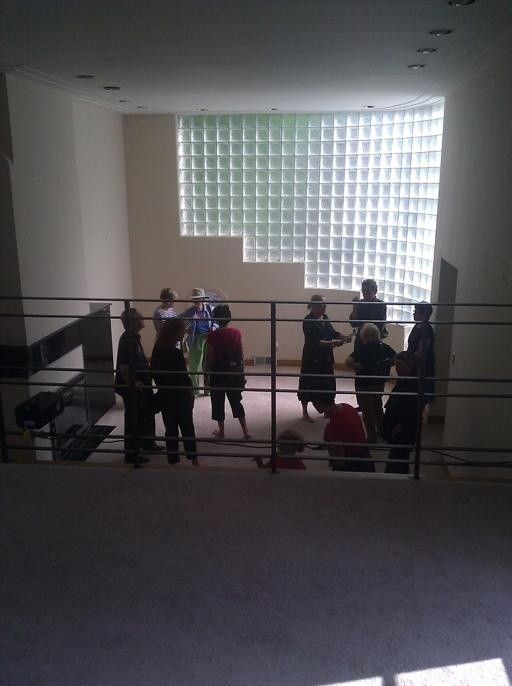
[189,288,210,299]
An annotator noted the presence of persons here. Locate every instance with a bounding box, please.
[345,323,397,443]
[251,428,307,469]
[382,351,417,474]
[310,386,375,473]
[297,294,353,422]
[349,279,386,351]
[407,300,434,419]
[113,286,251,468]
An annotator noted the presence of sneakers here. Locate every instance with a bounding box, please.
[125,455,150,463]
[143,443,165,451]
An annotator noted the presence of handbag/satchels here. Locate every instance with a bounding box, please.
[215,362,243,385]
